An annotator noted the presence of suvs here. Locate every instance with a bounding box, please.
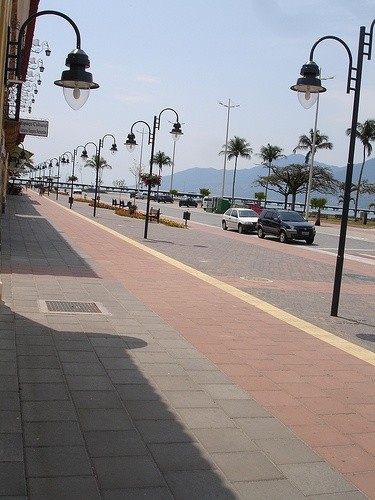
[257,208,316,246]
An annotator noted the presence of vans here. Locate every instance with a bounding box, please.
[202,195,229,211]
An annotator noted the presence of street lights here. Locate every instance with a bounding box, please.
[3,10,100,112]
[80,134,119,217]
[217,99,241,198]
[166,119,186,192]
[61,145,89,210]
[302,66,334,222]
[25,158,54,198]
[289,17,375,317]
[49,153,70,201]
[133,126,152,188]
[123,108,184,238]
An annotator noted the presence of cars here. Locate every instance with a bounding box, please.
[73,188,82,195]
[129,190,156,201]
[157,195,174,204]
[178,197,198,208]
[221,207,260,233]
